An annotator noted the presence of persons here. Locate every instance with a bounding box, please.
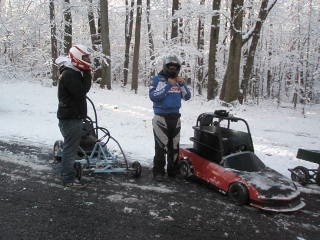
[57,46,93,189]
[150,55,191,185]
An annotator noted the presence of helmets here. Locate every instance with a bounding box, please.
[68,45,94,72]
[162,54,181,77]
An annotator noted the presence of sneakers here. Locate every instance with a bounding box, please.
[168,174,181,182]
[154,174,164,182]
[62,178,89,189]
